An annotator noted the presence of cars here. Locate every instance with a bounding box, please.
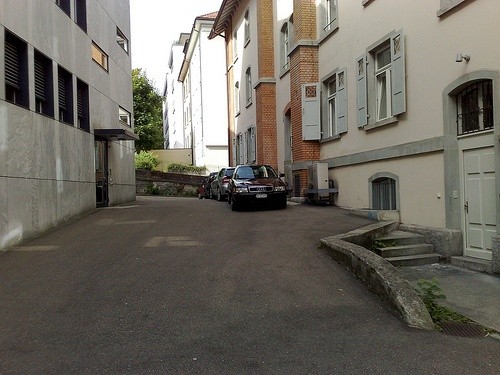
[204,172,219,198]
[226,165,287,211]
[198,184,205,199]
[210,167,235,200]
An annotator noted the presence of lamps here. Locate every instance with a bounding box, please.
[455,52,470,63]
